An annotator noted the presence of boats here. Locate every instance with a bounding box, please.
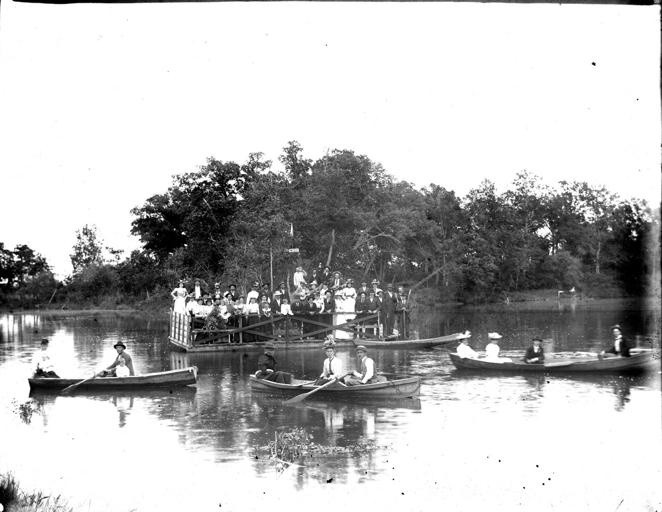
[249,374,421,398]
[28,365,199,393]
[449,348,662,372]
[354,332,463,348]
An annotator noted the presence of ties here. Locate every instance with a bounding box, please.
[329,358,332,374]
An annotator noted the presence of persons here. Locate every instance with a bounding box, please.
[600,324,631,358]
[101,341,135,376]
[340,345,378,386]
[33,338,59,378]
[169,264,412,342]
[257,342,291,383]
[317,341,340,384]
[458,337,480,360]
[485,331,514,362]
[523,335,545,364]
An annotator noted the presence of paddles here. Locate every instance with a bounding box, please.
[282,374,349,404]
[62,362,118,392]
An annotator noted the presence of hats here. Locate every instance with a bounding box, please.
[354,345,368,352]
[262,342,277,350]
[358,279,393,296]
[294,262,354,301]
[532,336,543,341]
[177,278,288,304]
[114,341,126,349]
[488,332,503,338]
[609,324,622,334]
[322,334,334,348]
[455,331,471,339]
